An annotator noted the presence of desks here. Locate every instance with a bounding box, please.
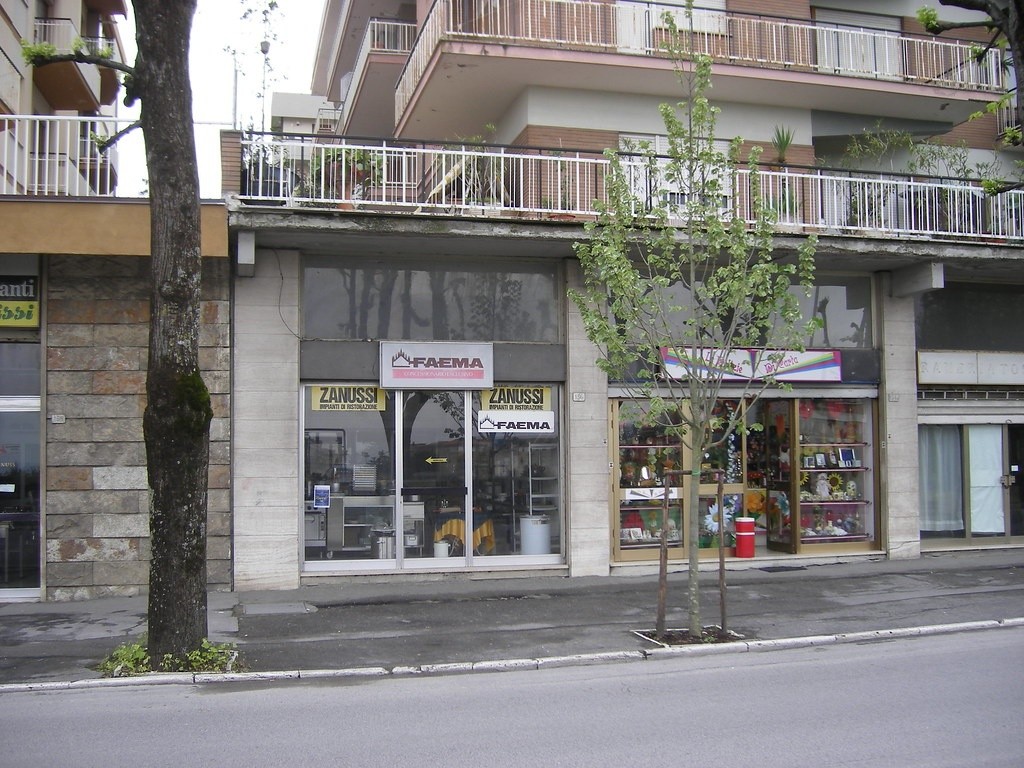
[432,513,495,557]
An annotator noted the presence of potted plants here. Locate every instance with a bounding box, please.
[768,123,795,172]
[373,30,384,48]
[242,123,302,204]
[543,138,577,220]
[443,122,504,215]
[313,145,384,210]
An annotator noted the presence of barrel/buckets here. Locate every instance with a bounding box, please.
[520,517,551,554]
[370,526,396,559]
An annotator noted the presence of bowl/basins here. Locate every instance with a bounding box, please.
[404,534,420,546]
[409,495,420,501]
[331,483,341,493]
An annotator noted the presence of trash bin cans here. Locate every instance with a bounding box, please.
[519,516,551,554]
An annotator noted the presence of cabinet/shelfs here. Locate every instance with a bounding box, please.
[306,439,558,559]
[612,397,883,561]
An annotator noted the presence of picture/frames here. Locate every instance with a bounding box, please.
[838,448,856,468]
[814,452,827,469]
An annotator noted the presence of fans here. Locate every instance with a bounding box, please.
[804,457,816,469]
